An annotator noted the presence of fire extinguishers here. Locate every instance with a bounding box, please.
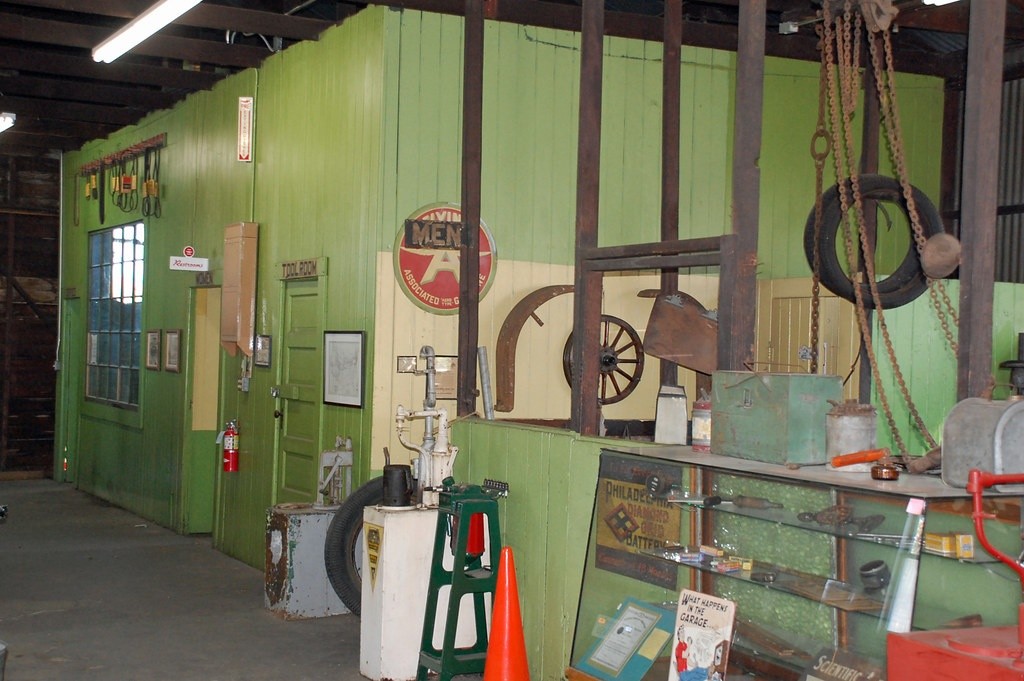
[223,421,240,472]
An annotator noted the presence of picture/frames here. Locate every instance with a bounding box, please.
[146,329,162,371]
[254,334,271,369]
[323,330,366,408]
[166,329,182,374]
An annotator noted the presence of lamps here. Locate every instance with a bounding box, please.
[92,0,203,63]
[0,112,16,132]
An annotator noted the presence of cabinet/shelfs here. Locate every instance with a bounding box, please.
[562,447,1024,681]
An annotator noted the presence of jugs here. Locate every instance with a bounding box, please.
[383,464,414,507]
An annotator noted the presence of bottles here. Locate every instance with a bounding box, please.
[859,559,891,593]
[691,400,713,453]
[824,398,878,473]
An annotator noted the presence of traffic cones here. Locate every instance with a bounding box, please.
[483,544,531,681]
